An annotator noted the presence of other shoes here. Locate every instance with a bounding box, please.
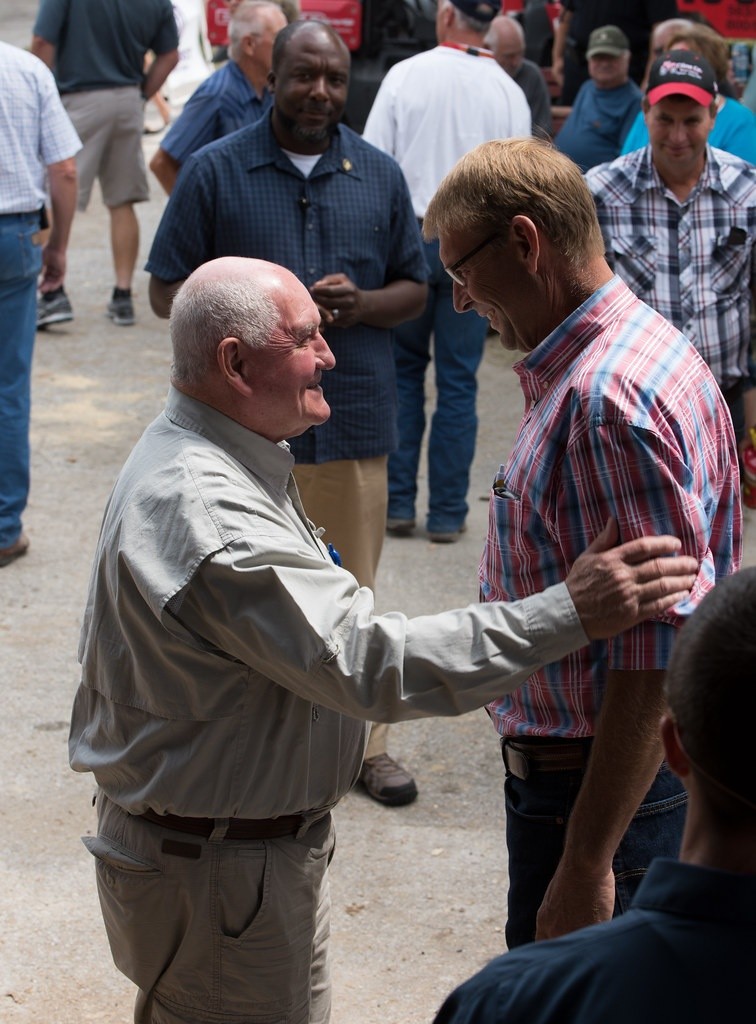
[0,533,29,568]
[386,518,416,532]
[430,521,468,543]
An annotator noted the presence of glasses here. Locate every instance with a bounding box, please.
[444,227,507,287]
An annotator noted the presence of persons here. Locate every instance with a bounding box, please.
[585,50,756,498]
[142,20,430,805]
[68,256,698,1024]
[481,0,756,174]
[0,42,83,570]
[423,137,743,952]
[432,567,756,1024]
[362,0,533,542]
[28,0,438,332]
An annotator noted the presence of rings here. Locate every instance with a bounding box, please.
[332,308,340,322]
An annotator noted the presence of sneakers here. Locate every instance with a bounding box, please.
[36,293,74,326]
[361,754,418,806]
[106,300,136,325]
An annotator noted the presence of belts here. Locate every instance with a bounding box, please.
[143,808,328,840]
[498,736,592,781]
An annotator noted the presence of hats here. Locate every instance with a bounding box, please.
[449,0,501,22]
[646,49,716,109]
[586,25,628,59]
